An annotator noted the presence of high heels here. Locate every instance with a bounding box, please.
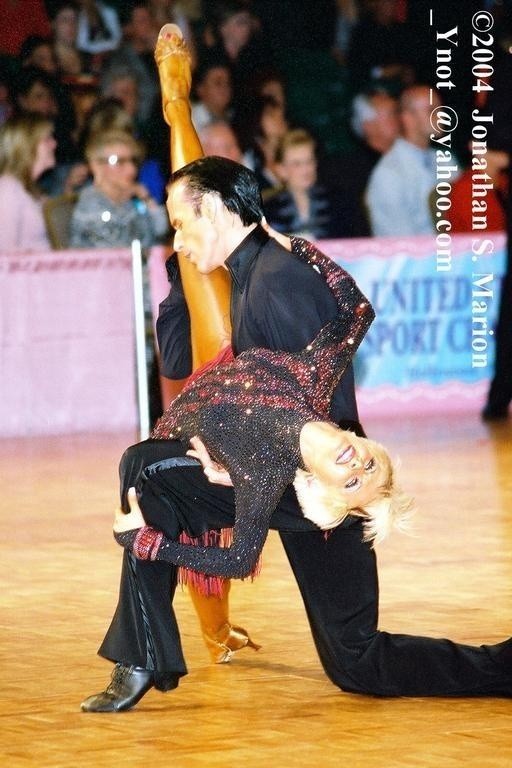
[78,662,179,710]
[203,625,261,663]
[153,24,194,127]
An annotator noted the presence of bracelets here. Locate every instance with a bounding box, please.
[132,525,152,559]
[139,529,158,559]
[150,530,164,561]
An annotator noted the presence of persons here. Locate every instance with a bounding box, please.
[0,1,512,257]
[81,155,510,715]
[468,139,510,422]
[115,22,418,666]
